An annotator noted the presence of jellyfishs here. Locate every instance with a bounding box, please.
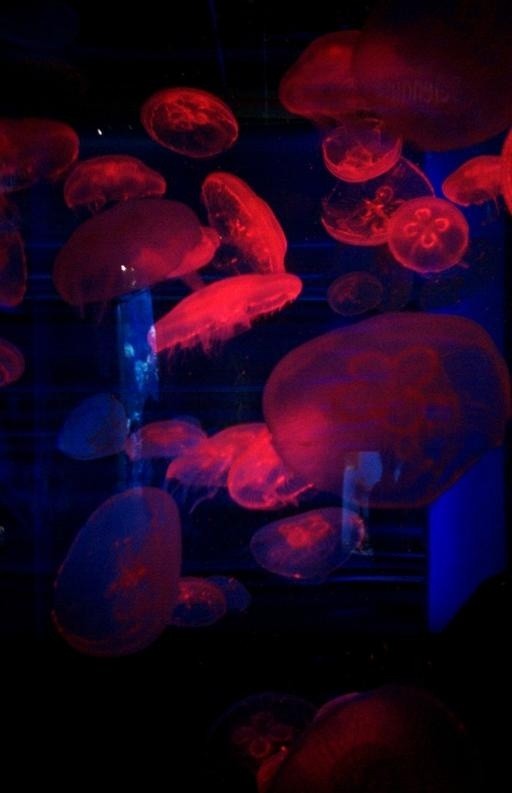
[0,15,512,662]
[235,683,451,793]
